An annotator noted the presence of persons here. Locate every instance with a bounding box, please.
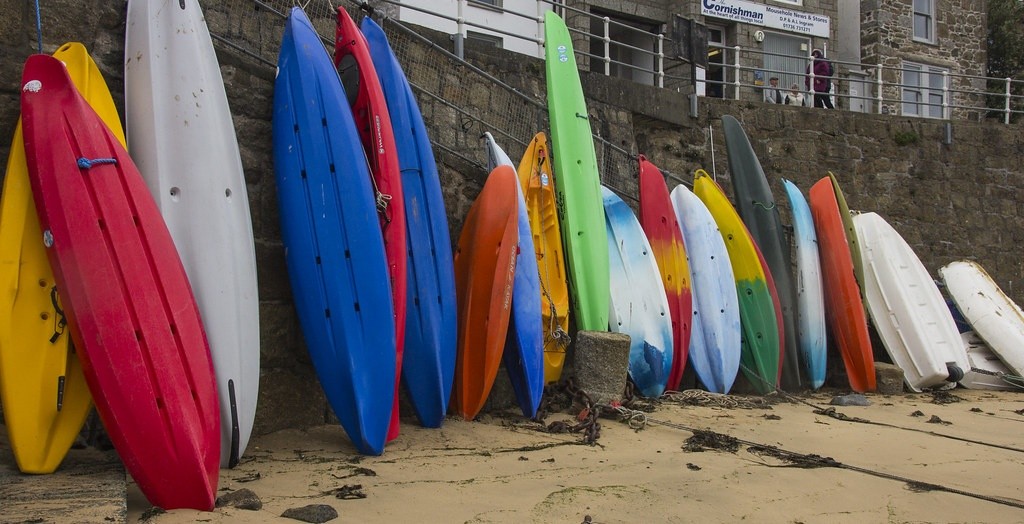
[765,77,783,104]
[785,83,806,106]
[806,49,834,109]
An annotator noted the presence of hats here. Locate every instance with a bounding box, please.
[792,83,798,88]
[770,77,778,81]
[812,49,821,53]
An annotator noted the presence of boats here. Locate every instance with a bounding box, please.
[542,10,611,333]
[0,41,131,475]
[479,131,544,418]
[719,113,804,388]
[636,153,693,394]
[447,165,519,423]
[935,259,1024,391]
[826,170,867,316]
[668,184,742,395]
[780,176,828,392]
[691,167,785,398]
[807,169,877,394]
[330,5,409,443]
[600,184,675,398]
[272,6,398,455]
[121,0,261,468]
[358,15,459,428]
[516,132,569,388]
[20,52,224,512]
[851,210,971,393]
